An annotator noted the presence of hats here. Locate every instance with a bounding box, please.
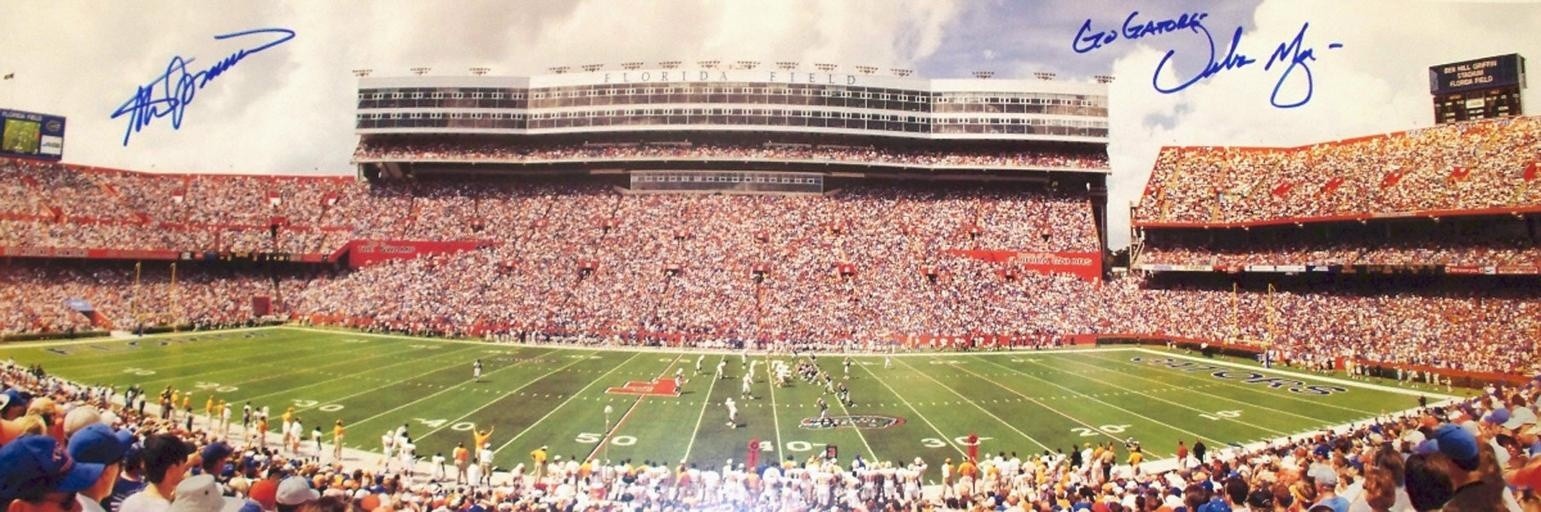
[203,441,234,462]
[0,388,143,464]
[144,434,197,466]
[1,435,107,500]
[167,476,441,512]
[1163,456,1271,511]
[1307,415,1479,512]
[1073,500,1113,512]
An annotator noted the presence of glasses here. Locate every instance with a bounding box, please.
[24,492,77,511]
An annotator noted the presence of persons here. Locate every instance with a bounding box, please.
[2,113,1541,373]
[1,350,1540,511]
